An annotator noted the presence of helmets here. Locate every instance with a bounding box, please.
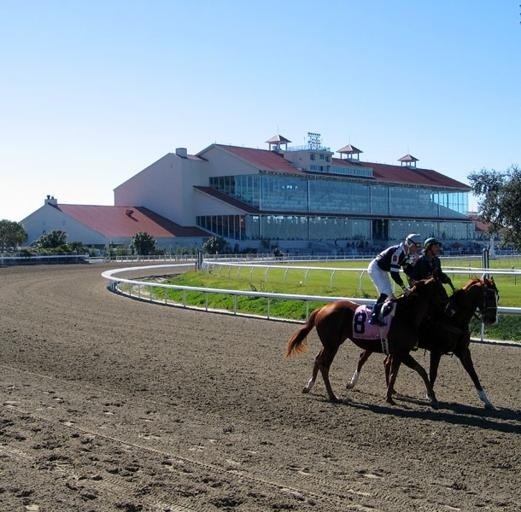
[423,237,442,248]
[406,233,423,248]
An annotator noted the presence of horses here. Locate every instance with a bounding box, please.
[287,272,500,414]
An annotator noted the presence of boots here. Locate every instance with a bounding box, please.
[368,292,389,327]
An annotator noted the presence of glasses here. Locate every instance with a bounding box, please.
[414,243,422,247]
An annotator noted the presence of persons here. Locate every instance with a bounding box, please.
[406,237,451,292]
[366,233,423,326]
